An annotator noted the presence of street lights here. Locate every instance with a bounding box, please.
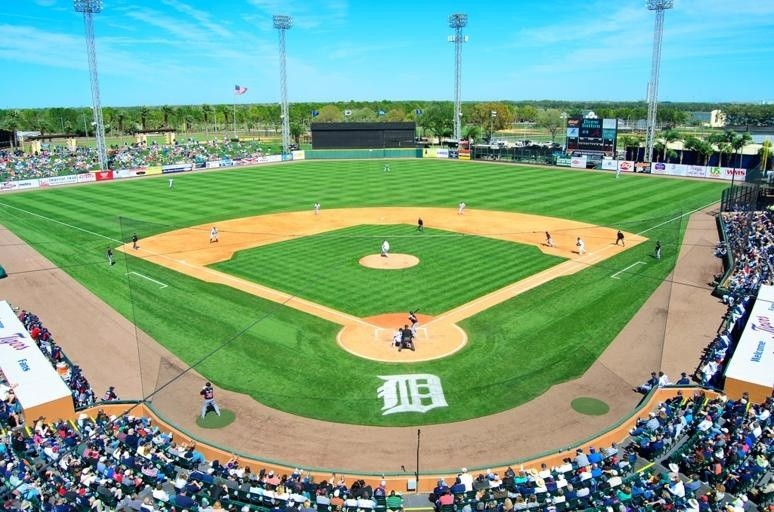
[489,109,497,143]
[643,0,674,163]
[74,0,111,172]
[273,14,292,154]
[559,111,568,149]
[447,11,468,143]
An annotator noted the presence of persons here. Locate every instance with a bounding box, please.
[388,309,428,353]
[382,162,390,174]
[2,129,311,189]
[207,228,220,241]
[577,237,586,257]
[1,374,404,512]
[457,201,467,215]
[415,217,424,232]
[691,212,773,376]
[427,374,769,510]
[311,203,321,213]
[544,230,558,247]
[103,244,113,266]
[379,237,391,259]
[2,297,94,376]
[130,233,138,248]
[613,229,625,248]
[654,239,663,260]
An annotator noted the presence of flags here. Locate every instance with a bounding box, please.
[234,84,247,95]
[311,107,423,118]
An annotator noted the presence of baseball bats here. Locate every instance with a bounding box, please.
[410,308,419,319]
